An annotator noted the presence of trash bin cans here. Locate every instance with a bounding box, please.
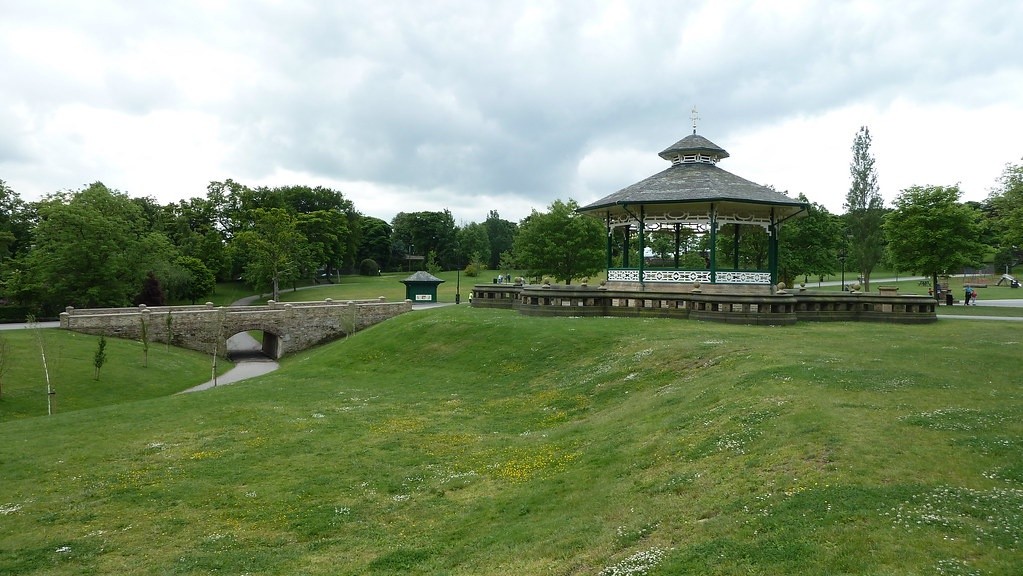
[946,294,953,306]
[493,278,497,284]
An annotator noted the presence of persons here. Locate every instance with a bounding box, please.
[468,291,473,303]
[963,285,977,305]
